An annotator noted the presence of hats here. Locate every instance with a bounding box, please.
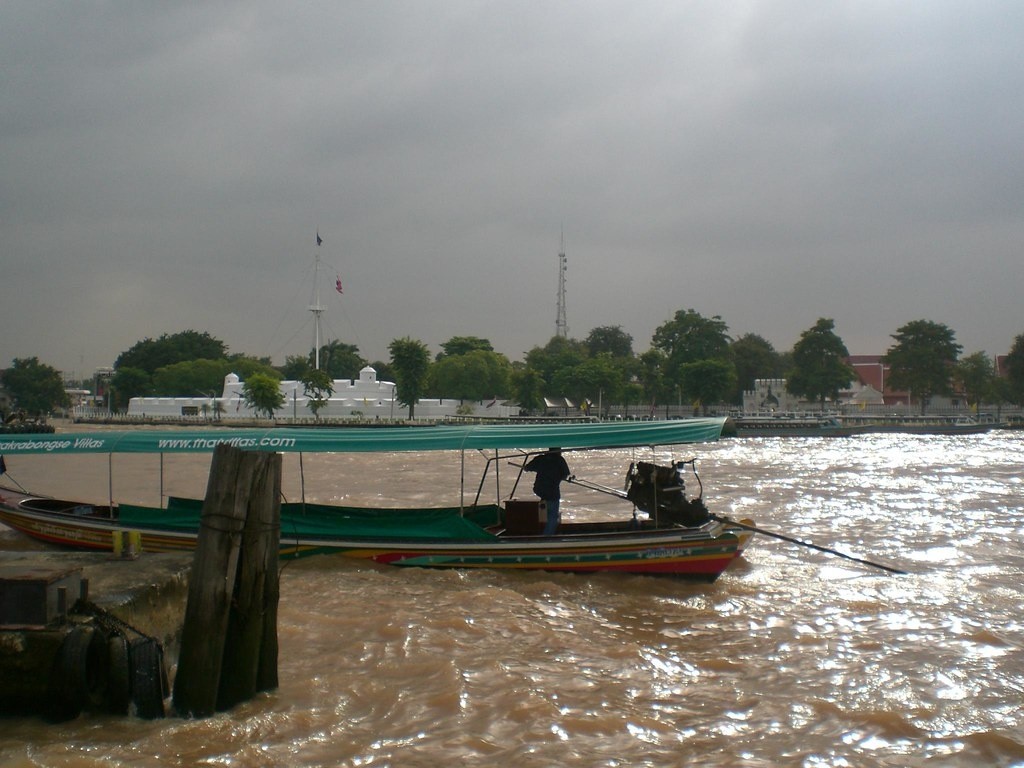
[549,448,561,453]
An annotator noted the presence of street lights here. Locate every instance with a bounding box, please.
[675,384,681,415]
[599,387,605,418]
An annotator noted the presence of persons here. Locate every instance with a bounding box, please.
[524,447,570,535]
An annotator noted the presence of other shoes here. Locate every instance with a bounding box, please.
[541,503,545,509]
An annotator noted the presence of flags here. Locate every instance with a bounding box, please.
[316,233,322,246]
[693,399,700,410]
[580,401,587,410]
[335,276,343,294]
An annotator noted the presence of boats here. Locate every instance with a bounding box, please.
[733,417,874,438]
[0,416,756,582]
[835,415,1008,434]
